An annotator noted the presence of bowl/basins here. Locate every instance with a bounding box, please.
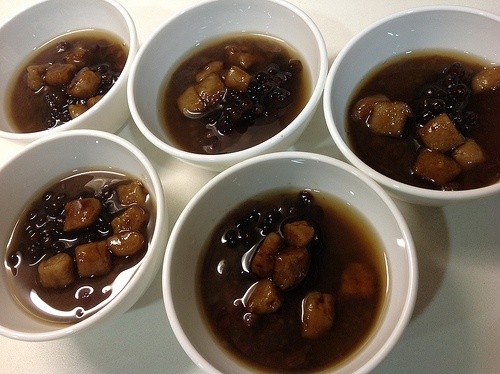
[323,4,500,209]
[0,0,141,144]
[161,149,417,374]
[127,0,329,176]
[0,129,170,343]
